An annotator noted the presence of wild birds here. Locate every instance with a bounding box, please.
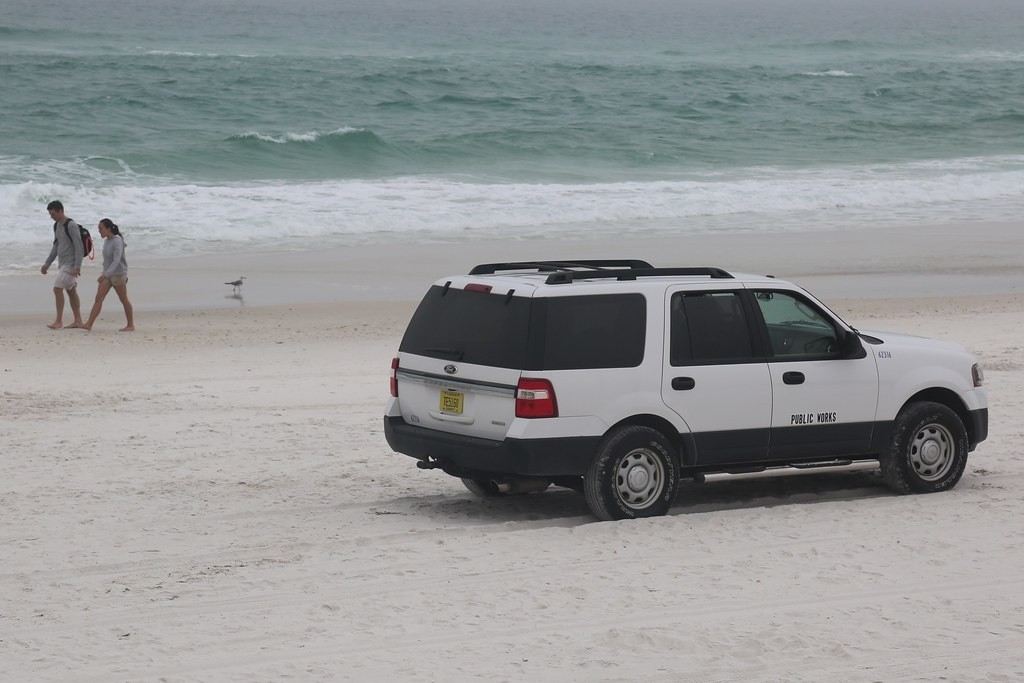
[224,276,247,292]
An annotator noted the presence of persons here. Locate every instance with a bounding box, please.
[76,218,136,333]
[40,200,85,329]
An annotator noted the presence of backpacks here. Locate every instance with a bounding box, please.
[53,218,96,262]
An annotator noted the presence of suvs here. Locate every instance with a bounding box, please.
[383,258,988,520]
